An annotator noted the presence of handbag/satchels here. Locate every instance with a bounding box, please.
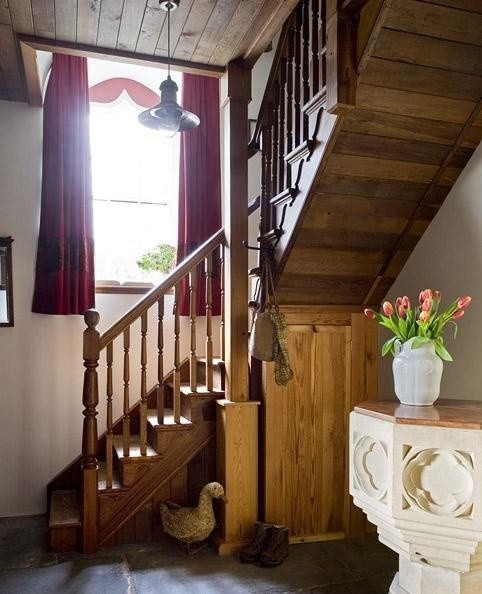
[249,300,273,362]
[266,303,294,386]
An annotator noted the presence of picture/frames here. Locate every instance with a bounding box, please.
[0,236,14,328]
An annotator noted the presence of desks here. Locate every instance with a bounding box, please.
[347,399,481,593]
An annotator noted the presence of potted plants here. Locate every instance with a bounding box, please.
[136,242,177,287]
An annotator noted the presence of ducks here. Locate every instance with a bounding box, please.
[159,481,229,557]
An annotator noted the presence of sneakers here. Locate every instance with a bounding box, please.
[239,521,273,564]
[259,525,290,567]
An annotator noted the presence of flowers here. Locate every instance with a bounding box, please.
[364,289,473,341]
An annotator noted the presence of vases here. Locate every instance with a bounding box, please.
[391,337,444,406]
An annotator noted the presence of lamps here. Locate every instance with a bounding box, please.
[138,0,201,138]
